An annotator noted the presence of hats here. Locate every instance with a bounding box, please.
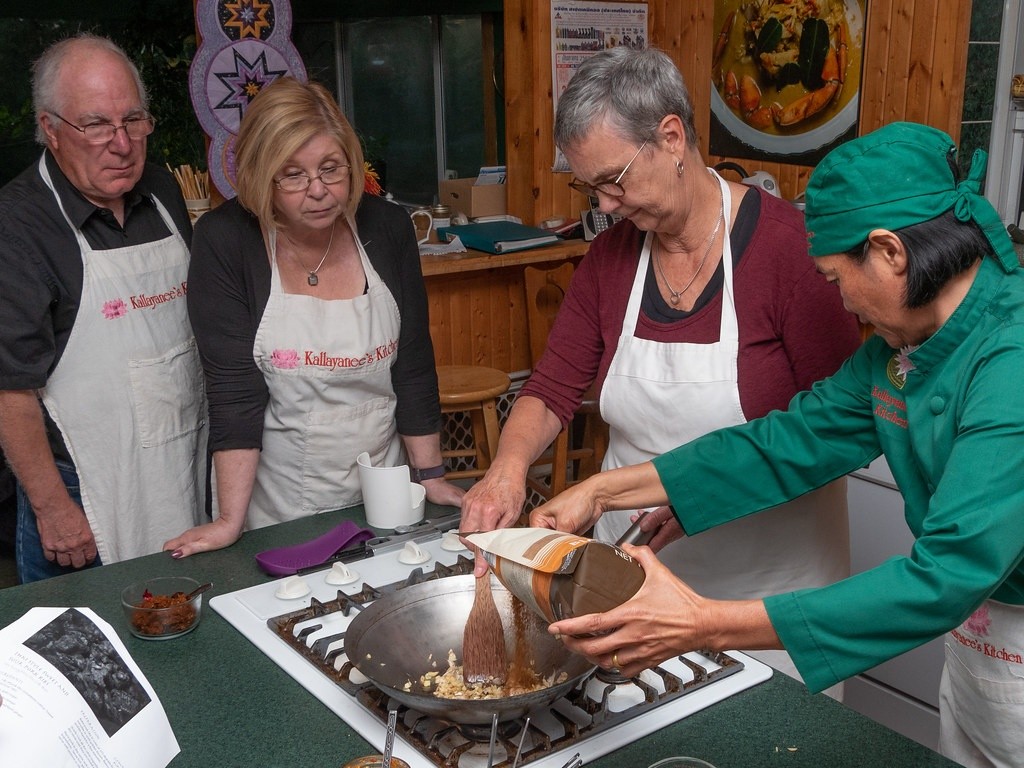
[805,123,1020,274]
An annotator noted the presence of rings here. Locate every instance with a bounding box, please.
[613,653,624,669]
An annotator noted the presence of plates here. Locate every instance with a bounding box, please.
[710,0,868,157]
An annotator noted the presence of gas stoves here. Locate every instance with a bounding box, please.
[208,529,774,768]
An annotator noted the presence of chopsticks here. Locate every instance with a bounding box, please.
[166,160,210,200]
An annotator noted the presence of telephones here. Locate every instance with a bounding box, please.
[580,194,624,241]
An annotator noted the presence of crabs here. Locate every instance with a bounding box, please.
[713,0,848,130]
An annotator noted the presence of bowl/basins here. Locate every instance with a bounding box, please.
[120,576,202,639]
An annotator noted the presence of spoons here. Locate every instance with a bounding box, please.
[170,583,213,600]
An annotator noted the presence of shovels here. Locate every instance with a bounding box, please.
[462,557,510,691]
[513,511,663,691]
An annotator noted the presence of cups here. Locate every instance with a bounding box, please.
[184,199,211,233]
[643,755,718,767]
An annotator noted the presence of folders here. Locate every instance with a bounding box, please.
[437,220,563,256]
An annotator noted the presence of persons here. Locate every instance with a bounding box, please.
[458,47,863,702]
[530,122,1024,768]
[0,35,208,584]
[162,77,466,559]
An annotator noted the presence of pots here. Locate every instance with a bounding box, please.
[344,573,598,724]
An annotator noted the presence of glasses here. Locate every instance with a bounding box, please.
[568,114,669,197]
[272,163,352,192]
[42,107,157,142]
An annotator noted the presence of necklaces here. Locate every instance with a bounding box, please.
[286,220,335,286]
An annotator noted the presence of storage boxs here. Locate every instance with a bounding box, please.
[438,177,506,217]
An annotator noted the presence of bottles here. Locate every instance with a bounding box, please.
[412,203,451,230]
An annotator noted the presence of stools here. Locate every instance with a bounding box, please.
[525,263,610,501]
[435,365,510,477]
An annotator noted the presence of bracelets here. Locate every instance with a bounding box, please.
[413,464,446,482]
